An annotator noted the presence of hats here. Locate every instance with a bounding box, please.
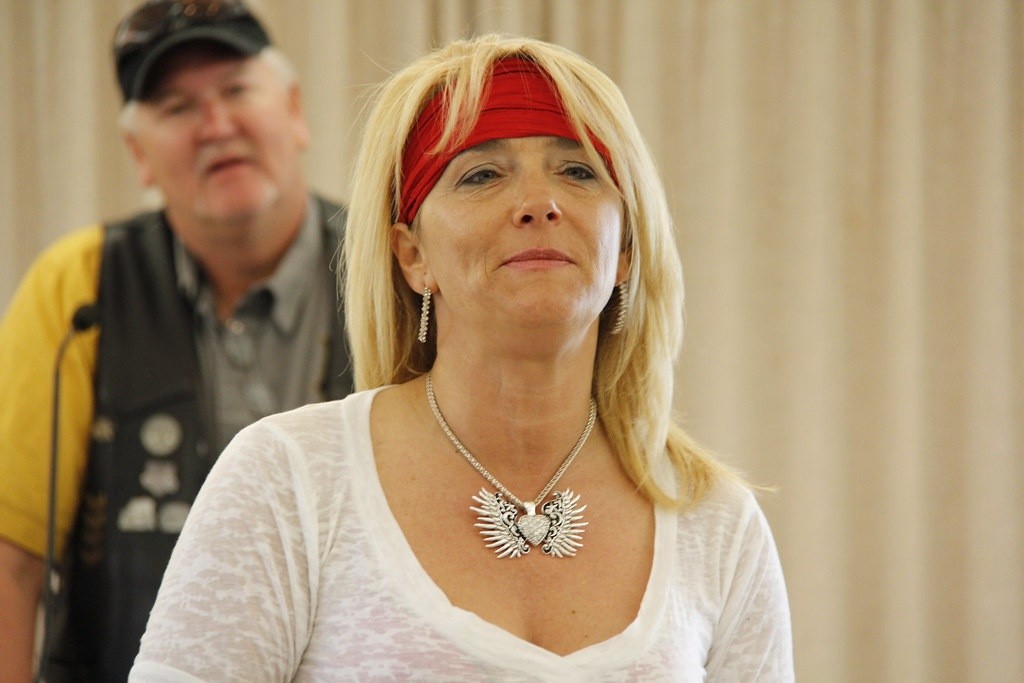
[114,0,269,101]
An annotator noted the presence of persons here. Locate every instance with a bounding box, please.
[0,0,355,683]
[126,35,796,683]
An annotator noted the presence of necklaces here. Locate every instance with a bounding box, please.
[427,370,597,558]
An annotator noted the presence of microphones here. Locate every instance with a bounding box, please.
[38,305,98,683]
